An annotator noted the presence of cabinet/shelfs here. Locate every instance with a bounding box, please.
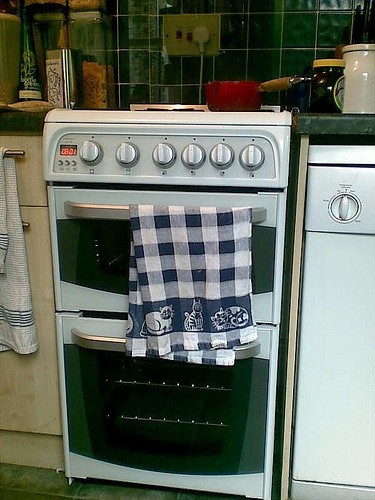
[0,135,65,471]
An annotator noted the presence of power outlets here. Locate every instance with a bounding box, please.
[160,14,222,58]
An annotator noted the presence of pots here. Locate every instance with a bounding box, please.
[204,76,292,113]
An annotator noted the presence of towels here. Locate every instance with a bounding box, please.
[125,204,258,366]
[0,147,38,354]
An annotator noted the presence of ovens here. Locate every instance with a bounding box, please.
[47,111,276,495]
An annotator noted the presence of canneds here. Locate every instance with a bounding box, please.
[309,57,347,116]
